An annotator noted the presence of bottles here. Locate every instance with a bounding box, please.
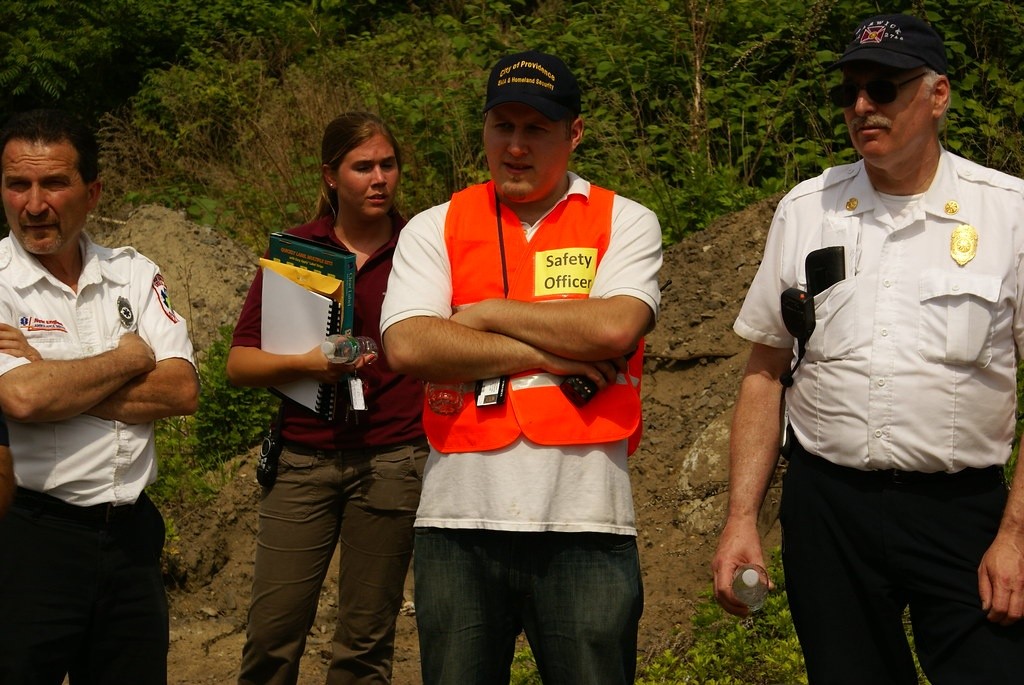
[323,335,379,366]
[732,564,769,612]
[426,383,465,414]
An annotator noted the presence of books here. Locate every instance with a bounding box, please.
[260,267,343,423]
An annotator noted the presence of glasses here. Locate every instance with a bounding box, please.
[829,70,927,109]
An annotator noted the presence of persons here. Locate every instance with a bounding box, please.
[711,11,1024,685]
[379,51,665,685]
[224,111,430,684]
[0,103,200,685]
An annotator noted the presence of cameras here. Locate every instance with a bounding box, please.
[257,434,284,486]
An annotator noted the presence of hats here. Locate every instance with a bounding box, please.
[482,48,580,123]
[826,11,948,79]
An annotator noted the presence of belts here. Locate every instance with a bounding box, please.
[12,488,114,530]
[792,442,1004,495]
[285,444,396,463]
